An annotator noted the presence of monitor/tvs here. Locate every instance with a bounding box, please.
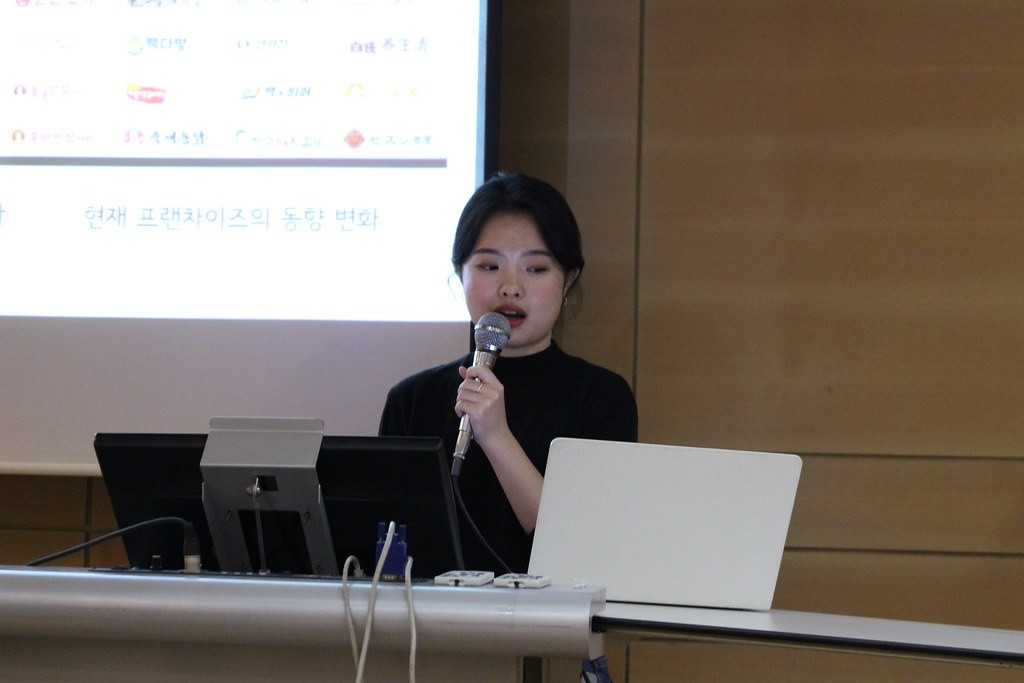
[93,432,465,584]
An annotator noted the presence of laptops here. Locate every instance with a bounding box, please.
[527,438,802,612]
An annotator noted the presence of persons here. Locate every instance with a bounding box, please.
[378,174,638,579]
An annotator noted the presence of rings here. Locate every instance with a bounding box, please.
[477,383,483,392]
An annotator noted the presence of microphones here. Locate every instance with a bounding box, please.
[451,312,511,475]
